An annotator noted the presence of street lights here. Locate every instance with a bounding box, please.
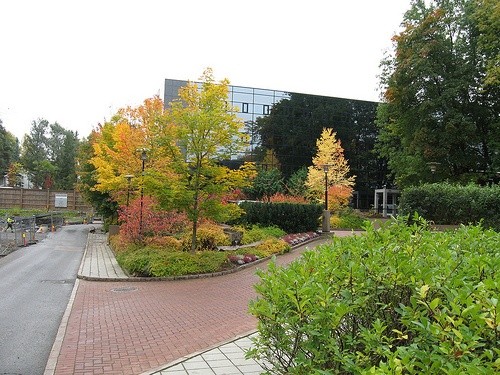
[319,161,335,233]
[123,174,134,235]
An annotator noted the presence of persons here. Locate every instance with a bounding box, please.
[3,214,15,233]
